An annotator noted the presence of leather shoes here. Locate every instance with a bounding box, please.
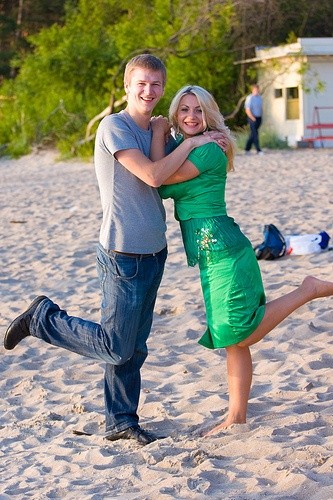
[3,295,50,351]
[106,426,168,443]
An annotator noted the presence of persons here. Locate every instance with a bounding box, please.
[149,86,332,437]
[244,84,264,157]
[3,55,228,444]
